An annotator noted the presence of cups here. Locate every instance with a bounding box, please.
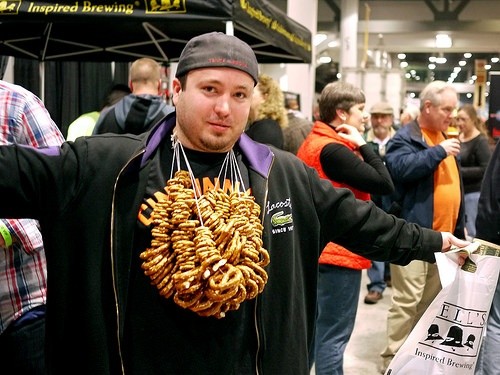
[446,122,459,138]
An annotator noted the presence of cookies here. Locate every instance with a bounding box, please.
[139,171,270,318]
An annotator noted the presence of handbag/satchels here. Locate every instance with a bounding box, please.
[385,244,500,375]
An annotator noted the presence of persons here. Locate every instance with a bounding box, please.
[278,98,312,157]
[0,81,65,375]
[92,58,175,136]
[65,83,130,143]
[295,82,392,375]
[246,72,288,151]
[365,80,500,375]
[0,32,470,375]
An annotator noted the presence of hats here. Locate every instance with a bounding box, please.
[175,31,257,87]
[369,104,394,114]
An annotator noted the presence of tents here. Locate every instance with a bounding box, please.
[0,0,313,108]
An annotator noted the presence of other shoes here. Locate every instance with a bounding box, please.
[364,289,382,303]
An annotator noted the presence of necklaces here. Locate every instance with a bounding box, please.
[136,132,269,320]
